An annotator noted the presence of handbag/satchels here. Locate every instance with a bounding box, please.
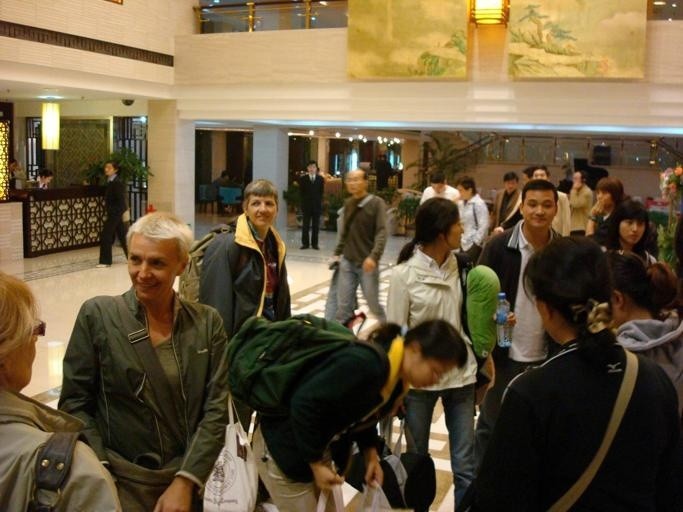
[475,349,496,407]
[339,412,437,512]
[201,392,260,511]
[98,444,201,511]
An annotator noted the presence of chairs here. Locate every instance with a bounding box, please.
[195,171,422,236]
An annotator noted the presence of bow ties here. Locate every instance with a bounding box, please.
[42,184,45,190]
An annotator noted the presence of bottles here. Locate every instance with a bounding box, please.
[494,292,514,352]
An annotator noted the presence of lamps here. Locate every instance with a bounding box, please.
[469,0,511,31]
[38,96,61,152]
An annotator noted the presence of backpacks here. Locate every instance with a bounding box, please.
[223,314,358,420]
[178,220,240,306]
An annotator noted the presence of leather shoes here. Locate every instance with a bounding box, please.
[312,245,320,250]
[299,243,310,250]
[96,263,113,268]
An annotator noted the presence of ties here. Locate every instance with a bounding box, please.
[311,173,314,183]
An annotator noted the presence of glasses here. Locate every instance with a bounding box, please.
[28,316,47,338]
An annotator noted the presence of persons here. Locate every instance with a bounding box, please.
[92,160,133,268]
[24,166,54,191]
[8,158,18,189]
[297,161,322,250]
[203,170,236,215]
[195,176,295,502]
[246,315,468,511]
[0,269,125,511]
[55,210,237,511]
